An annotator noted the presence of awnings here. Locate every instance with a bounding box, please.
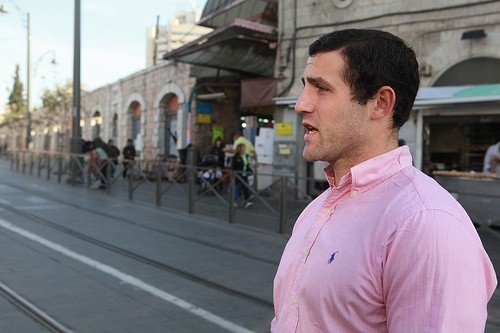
[411,85,499,107]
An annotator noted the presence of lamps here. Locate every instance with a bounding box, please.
[460,30,487,39]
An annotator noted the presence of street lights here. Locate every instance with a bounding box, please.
[0,1,31,151]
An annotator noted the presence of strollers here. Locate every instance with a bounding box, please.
[199,154,222,196]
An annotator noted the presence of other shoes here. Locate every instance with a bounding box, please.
[234,203,237,207]
[244,202,252,209]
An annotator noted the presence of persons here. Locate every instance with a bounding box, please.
[122,138,136,180]
[482,140,500,177]
[208,136,226,167]
[81,138,121,190]
[228,142,255,207]
[398,138,415,167]
[268,28,498,333]
[223,131,259,208]
[202,167,216,192]
